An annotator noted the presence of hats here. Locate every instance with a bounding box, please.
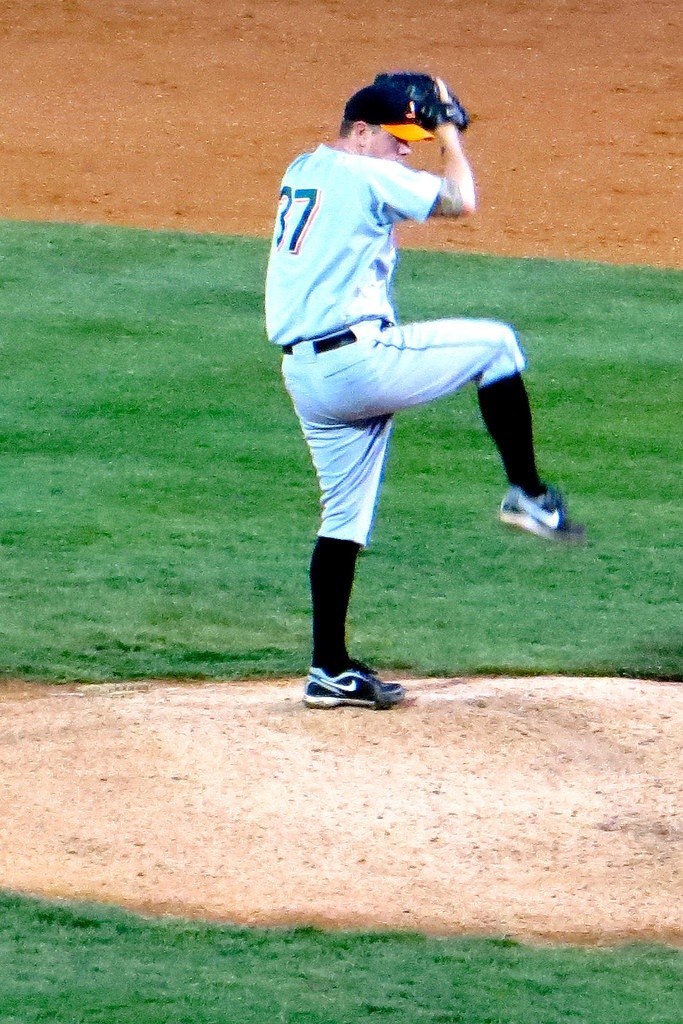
[344,85,437,141]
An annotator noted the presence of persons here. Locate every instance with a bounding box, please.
[251,69,593,713]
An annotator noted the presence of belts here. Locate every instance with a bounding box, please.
[281,319,393,354]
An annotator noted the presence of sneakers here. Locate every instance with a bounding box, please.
[302,666,405,711]
[501,486,586,544]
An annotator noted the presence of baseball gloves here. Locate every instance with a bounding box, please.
[373,70,469,133]
